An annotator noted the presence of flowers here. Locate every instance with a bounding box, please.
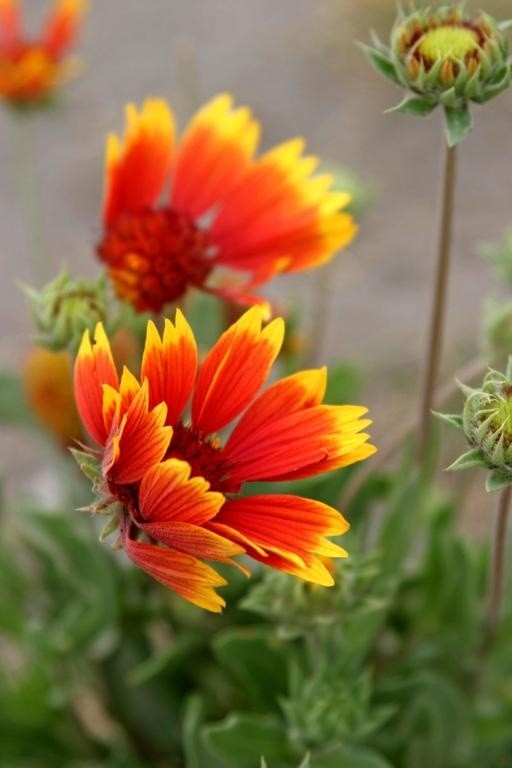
[1,2,512,766]
